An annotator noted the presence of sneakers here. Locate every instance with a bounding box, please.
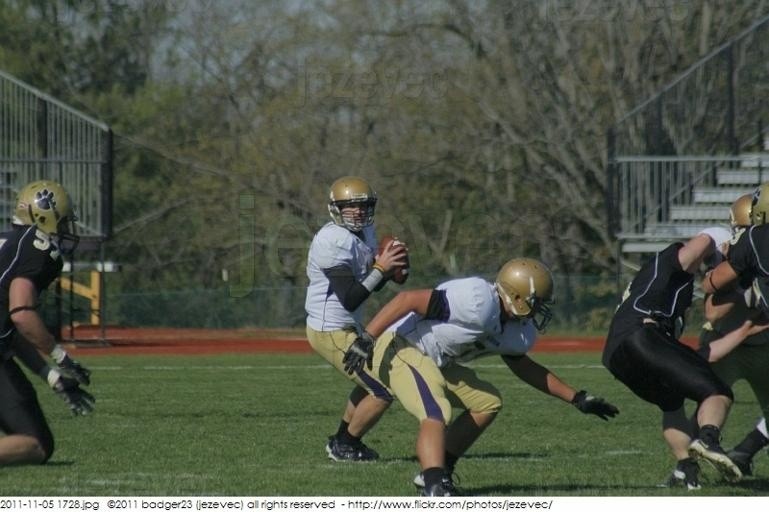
[688,428,754,482]
[413,468,464,497]
[666,459,702,489]
[325,433,378,462]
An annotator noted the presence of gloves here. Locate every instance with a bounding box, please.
[49,348,95,416]
[342,333,375,375]
[572,390,619,421]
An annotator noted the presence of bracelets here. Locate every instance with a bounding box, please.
[372,263,386,274]
[38,364,62,389]
[48,342,66,364]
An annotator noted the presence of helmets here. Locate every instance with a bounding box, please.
[728,180,769,230]
[10,181,79,255]
[495,257,556,332]
[327,176,376,230]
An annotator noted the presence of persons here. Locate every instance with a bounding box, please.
[602,181,769,490]
[0,179,96,467]
[304,176,409,462]
[342,258,619,496]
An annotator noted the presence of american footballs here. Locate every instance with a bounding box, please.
[380,235,410,284]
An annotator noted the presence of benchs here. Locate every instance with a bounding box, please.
[617,120,768,267]
[0,161,99,238]
